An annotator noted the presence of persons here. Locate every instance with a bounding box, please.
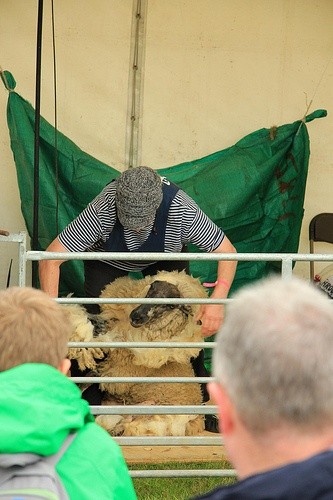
[0,286,138,500]
[39,165,237,433]
[188,274,333,500]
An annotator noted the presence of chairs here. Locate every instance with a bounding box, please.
[308,213,333,300]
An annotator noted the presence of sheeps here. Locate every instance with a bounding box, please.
[63,269,206,438]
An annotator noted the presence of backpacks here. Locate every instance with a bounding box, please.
[0,433,75,500]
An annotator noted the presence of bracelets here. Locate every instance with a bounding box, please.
[203,279,231,290]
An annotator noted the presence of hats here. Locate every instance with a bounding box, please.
[114,166,162,231]
